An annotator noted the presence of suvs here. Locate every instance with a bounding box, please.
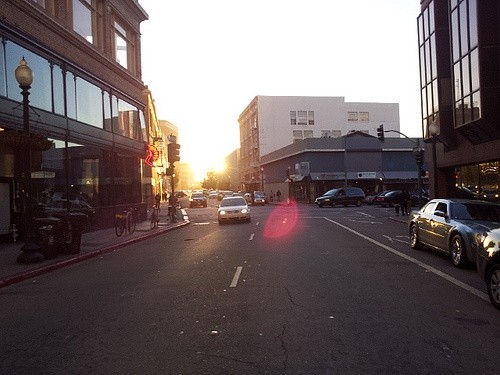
[314,187,366,208]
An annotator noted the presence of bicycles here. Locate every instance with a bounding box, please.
[115,207,138,237]
[150,206,161,229]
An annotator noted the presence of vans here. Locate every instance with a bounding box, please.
[253,191,266,206]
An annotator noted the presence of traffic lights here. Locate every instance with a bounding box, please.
[376,123,385,142]
[168,143,181,162]
[415,151,421,166]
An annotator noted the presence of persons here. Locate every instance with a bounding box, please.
[155,192,167,208]
[269,190,281,202]
[393,189,409,216]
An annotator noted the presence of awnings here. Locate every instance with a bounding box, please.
[383,171,425,179]
[293,176,308,182]
[310,172,384,180]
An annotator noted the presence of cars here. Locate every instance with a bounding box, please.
[50,198,96,221]
[366,190,390,205]
[189,193,208,208]
[244,193,251,203]
[374,190,430,208]
[176,188,244,201]
[477,229,500,309]
[407,199,500,267]
[217,197,251,224]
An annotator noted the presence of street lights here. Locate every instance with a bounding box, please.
[343,130,356,186]
[261,167,265,191]
[228,176,231,190]
[14,55,43,265]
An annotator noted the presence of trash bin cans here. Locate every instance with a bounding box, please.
[32,217,62,259]
[48,212,86,254]
